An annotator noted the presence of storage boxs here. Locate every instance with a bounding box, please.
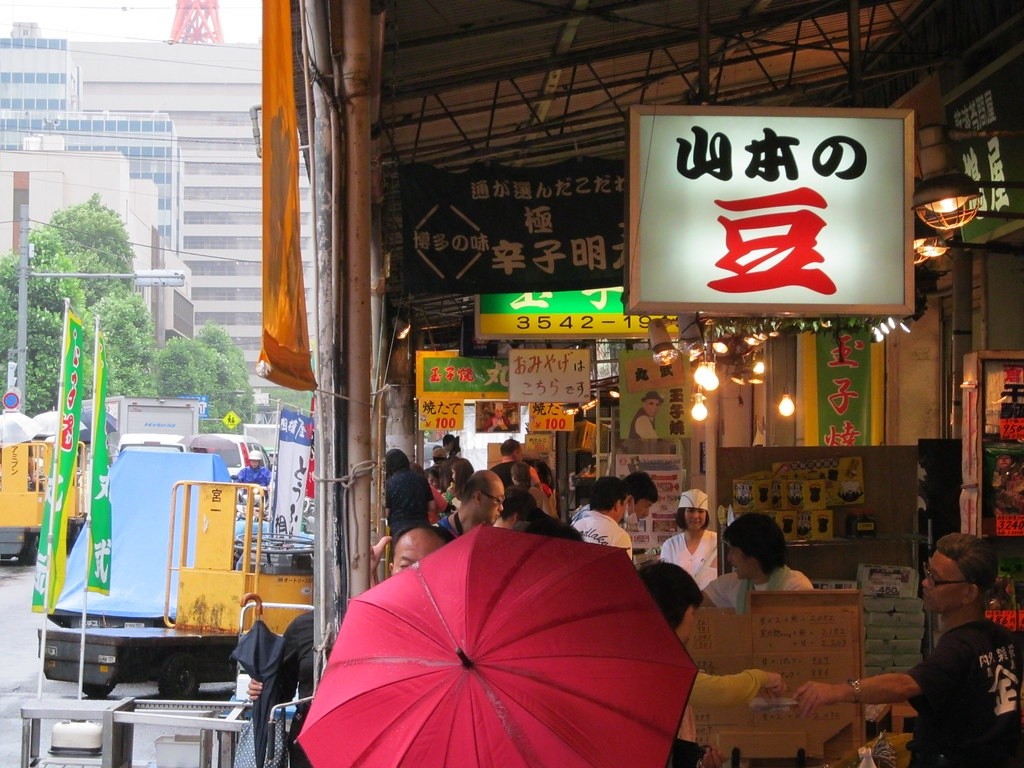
[154,734,201,768]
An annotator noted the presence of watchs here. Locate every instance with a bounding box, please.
[848,678,863,703]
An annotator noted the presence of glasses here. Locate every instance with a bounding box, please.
[922,560,973,587]
[473,490,505,504]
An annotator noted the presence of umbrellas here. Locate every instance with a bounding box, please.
[231,594,287,768]
[298,522,699,768]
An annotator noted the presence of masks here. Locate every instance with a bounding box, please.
[624,496,640,525]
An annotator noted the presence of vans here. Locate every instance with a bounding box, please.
[112,432,271,482]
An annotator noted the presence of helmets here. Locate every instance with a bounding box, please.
[249,450,263,460]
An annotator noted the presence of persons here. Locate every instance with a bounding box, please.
[409,433,474,525]
[490,439,584,542]
[381,448,435,536]
[659,488,718,591]
[792,532,1024,768]
[370,523,457,589]
[29,445,44,491]
[233,449,272,487]
[630,391,664,439]
[248,607,347,768]
[637,562,789,768]
[432,470,506,541]
[485,403,512,432]
[697,512,813,609]
[570,469,658,563]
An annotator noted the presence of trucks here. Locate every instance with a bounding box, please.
[0,440,86,562]
[79,395,201,449]
[35,478,314,700]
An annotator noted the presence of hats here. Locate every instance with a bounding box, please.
[433,448,447,458]
[678,489,709,510]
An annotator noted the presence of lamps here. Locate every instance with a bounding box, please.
[917,236,950,257]
[909,172,983,233]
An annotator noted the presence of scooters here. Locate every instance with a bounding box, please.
[237,486,268,521]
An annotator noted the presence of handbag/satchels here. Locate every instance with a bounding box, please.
[232,708,289,768]
